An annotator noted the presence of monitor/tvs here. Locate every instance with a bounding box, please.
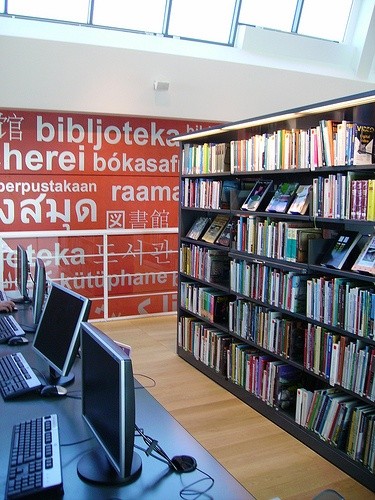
[7,244,32,302]
[18,258,47,332]
[31,282,92,387]
[78,322,143,487]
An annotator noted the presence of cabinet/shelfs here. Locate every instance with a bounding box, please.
[174,88,375,494]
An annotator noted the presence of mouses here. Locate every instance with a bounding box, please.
[8,337,30,345]
[41,385,67,397]
[7,306,18,312]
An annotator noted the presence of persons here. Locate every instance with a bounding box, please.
[0,301,15,313]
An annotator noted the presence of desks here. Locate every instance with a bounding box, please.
[0,293,257,500]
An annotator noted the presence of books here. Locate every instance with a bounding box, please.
[178,119,375,474]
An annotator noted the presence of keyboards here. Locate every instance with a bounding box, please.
[0,352,41,399]
[0,315,26,343]
[5,414,63,500]
